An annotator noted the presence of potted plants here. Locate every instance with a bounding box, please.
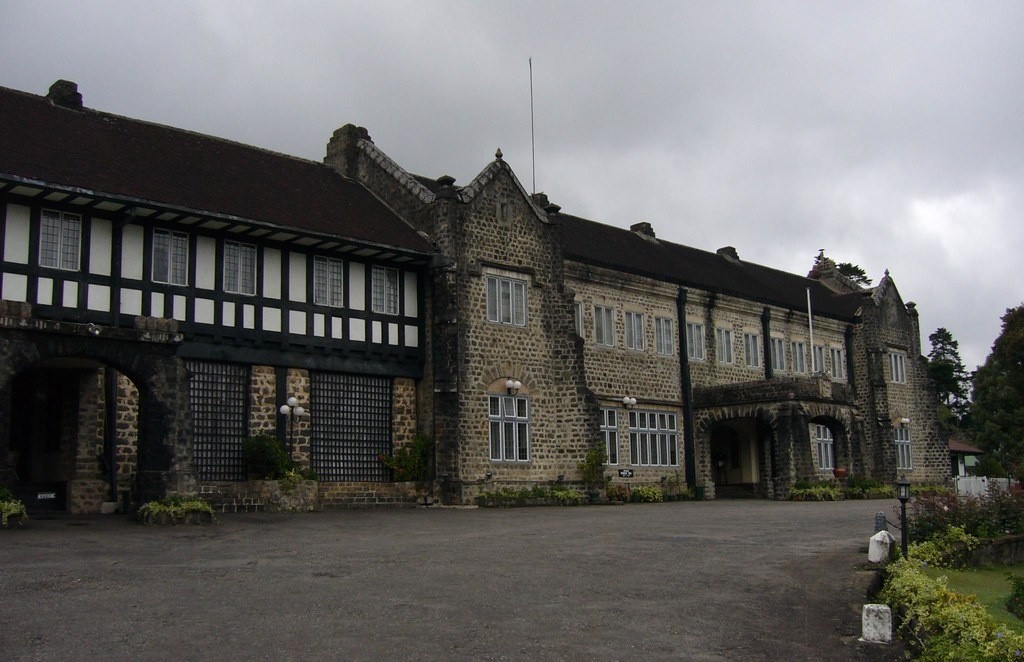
[131,491,215,527]
[474,486,584,507]
[0,487,28,530]
[240,433,322,515]
[599,470,703,506]
[578,440,607,501]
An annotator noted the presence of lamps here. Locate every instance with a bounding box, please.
[623,397,637,411]
[505,380,521,396]
[895,473,912,503]
[88,323,103,336]
[901,417,910,428]
[280,397,305,424]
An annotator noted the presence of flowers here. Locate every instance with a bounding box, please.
[379,433,429,482]
